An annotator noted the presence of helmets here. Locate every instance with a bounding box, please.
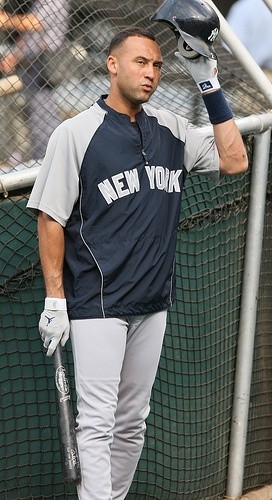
[149,0,221,58]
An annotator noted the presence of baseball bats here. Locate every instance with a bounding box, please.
[50,340,82,485]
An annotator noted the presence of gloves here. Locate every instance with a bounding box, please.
[173,51,220,95]
[39,298,70,357]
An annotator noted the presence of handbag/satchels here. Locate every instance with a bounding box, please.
[41,49,76,89]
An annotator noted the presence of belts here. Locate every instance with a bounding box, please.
[16,57,41,68]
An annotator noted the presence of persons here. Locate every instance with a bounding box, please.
[26,26,248,500]
[0,0,70,164]
